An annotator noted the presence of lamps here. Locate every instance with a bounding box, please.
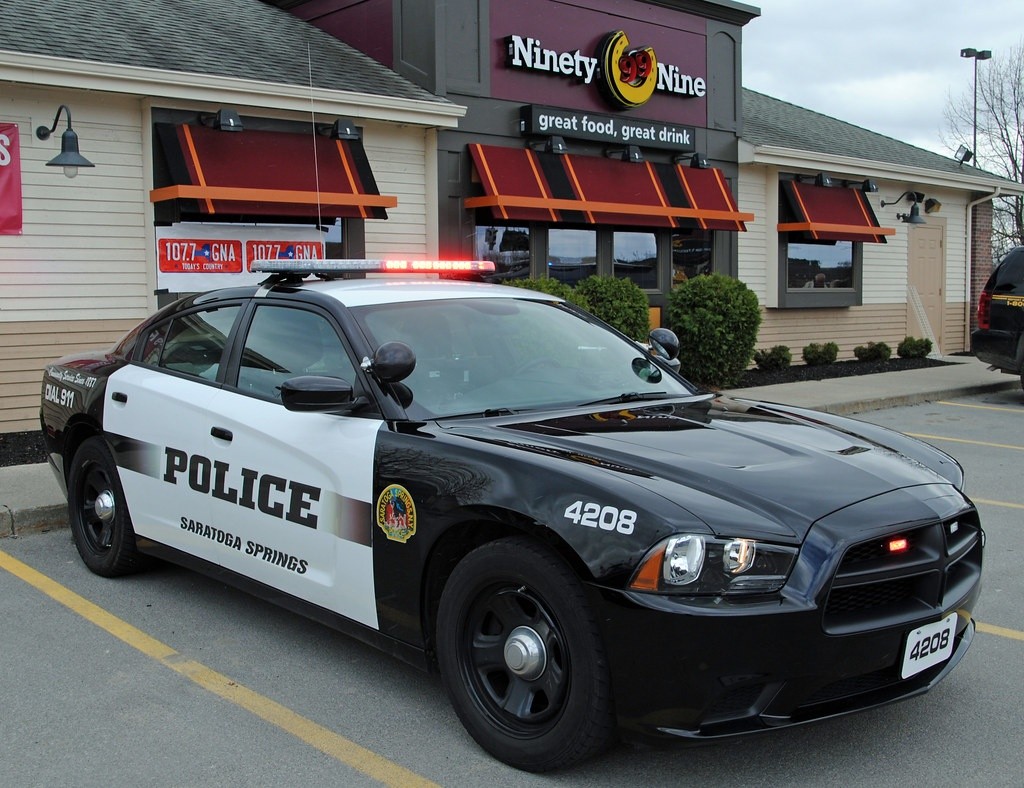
[200,108,245,132]
[881,190,927,224]
[954,144,973,166]
[36,104,95,178]
[672,152,711,169]
[842,178,878,192]
[925,197,941,213]
[602,145,644,162]
[526,135,568,153]
[797,172,833,187]
[314,118,359,139]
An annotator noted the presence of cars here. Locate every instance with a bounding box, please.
[971,242,1024,391]
[39,256,986,777]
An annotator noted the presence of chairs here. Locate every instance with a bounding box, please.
[242,309,323,395]
[397,310,473,409]
